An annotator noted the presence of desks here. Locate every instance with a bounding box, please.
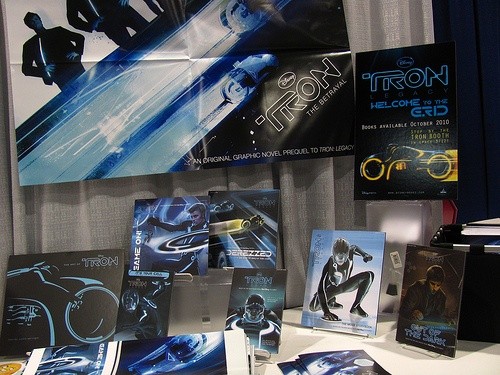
[253,305,500,375]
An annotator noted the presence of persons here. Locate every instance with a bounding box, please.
[21,12,87,93]
[308,238,375,322]
[146,203,209,277]
[66,0,150,48]
[225,294,282,354]
[400,264,447,321]
